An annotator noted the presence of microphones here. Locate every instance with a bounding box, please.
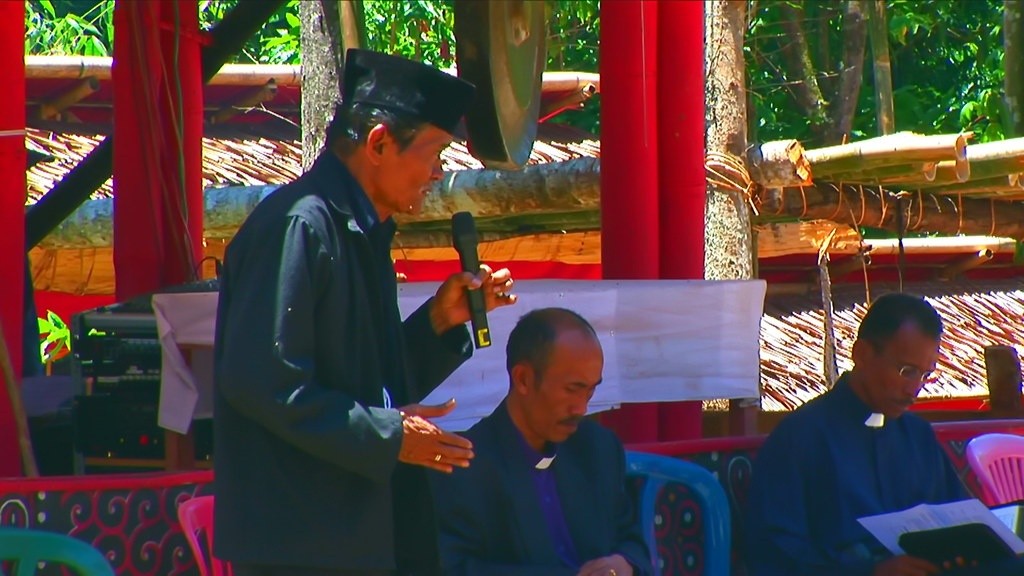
[451,212,491,348]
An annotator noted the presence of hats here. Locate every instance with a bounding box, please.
[343,48,477,140]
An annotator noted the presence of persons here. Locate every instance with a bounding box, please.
[737,294,1024,576]
[421,306,654,576]
[209,48,518,575]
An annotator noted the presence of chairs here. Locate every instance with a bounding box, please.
[0,526,116,576]
[624,449,732,576]
[967,433,1024,503]
[179,495,236,576]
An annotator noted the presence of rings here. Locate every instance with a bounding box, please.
[610,568,618,576]
[435,452,441,462]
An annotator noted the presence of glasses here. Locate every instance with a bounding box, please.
[865,338,938,384]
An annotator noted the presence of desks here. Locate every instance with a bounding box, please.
[153,277,768,474]
